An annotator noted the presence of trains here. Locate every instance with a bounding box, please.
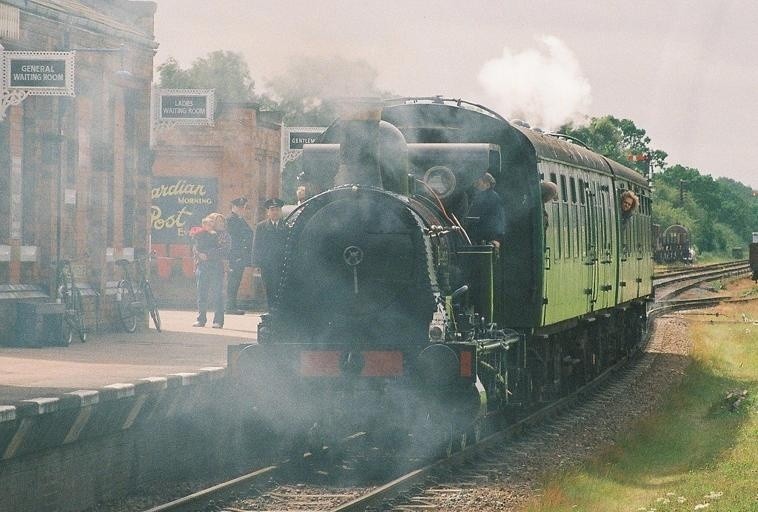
[224,96,656,460]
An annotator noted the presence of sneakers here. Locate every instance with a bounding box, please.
[211,323,224,329]
[224,308,246,316]
[191,322,206,327]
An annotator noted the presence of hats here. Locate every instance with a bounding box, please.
[230,197,247,204]
[264,198,284,208]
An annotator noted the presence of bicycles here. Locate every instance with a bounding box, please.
[116,247,162,333]
[51,251,90,345]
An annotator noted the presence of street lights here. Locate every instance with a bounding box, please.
[52,24,135,303]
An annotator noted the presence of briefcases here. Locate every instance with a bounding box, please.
[251,267,265,301]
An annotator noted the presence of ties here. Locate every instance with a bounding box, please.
[273,222,278,229]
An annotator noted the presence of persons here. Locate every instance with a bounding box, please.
[191,220,233,277]
[621,191,638,220]
[192,214,231,329]
[541,181,558,227]
[463,171,506,258]
[224,197,253,315]
[253,199,289,312]
